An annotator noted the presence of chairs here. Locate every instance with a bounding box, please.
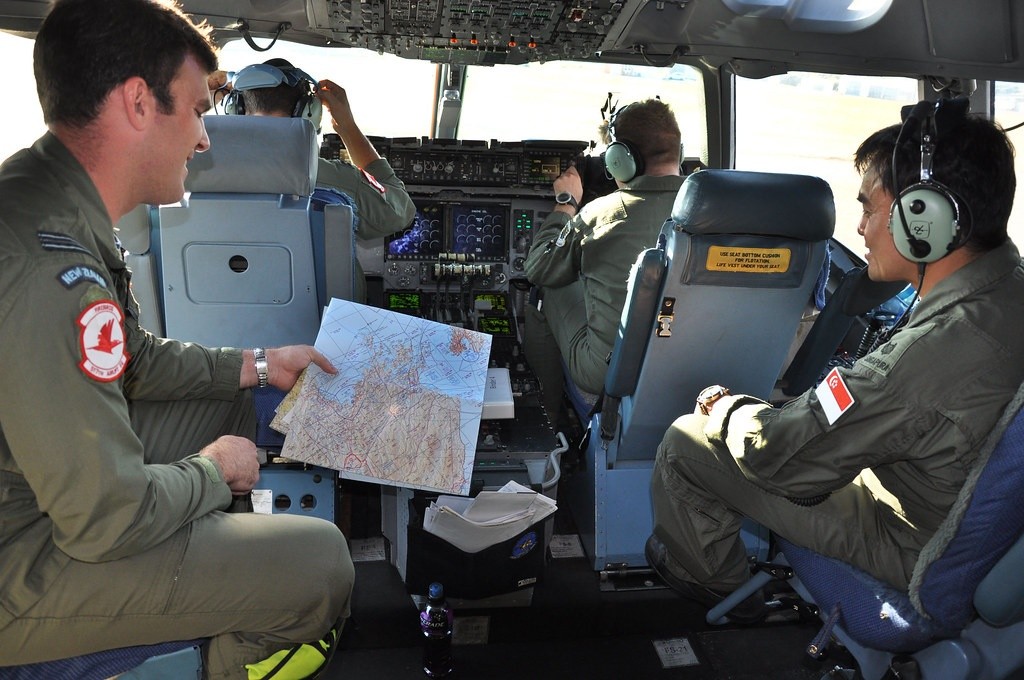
[0,637,206,680]
[113,116,357,525]
[524,169,836,592]
[705,383,1024,680]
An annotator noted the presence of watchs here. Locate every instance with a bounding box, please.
[556,191,577,209]
[698,385,732,415]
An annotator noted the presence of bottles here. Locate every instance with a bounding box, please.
[420,583,454,678]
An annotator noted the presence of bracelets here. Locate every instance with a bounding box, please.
[253,347,268,388]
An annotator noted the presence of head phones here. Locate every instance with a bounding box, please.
[604,105,645,183]
[887,97,973,264]
[225,64,323,135]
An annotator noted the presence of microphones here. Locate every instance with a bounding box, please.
[891,100,932,259]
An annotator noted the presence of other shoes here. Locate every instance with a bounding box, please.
[645,533,766,625]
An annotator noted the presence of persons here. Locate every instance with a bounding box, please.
[526,101,686,434]
[1,0,354,680]
[604,113,1024,631]
[211,58,416,304]
